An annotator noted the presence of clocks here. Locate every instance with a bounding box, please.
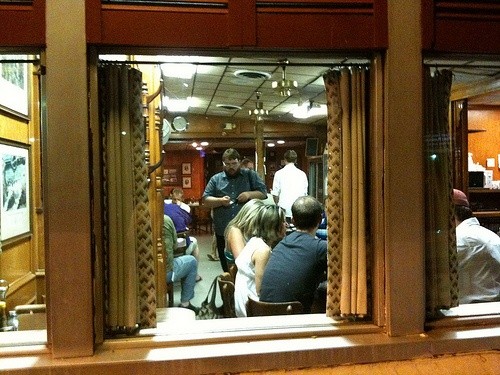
[172,116,188,131]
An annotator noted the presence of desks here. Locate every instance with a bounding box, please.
[187,202,205,230]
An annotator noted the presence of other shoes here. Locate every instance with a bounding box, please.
[207,253,220,261]
[178,301,201,315]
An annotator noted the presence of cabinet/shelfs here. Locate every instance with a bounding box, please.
[468,189,500,234]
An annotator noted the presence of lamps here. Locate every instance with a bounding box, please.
[233,70,272,80]
[248,91,268,126]
[216,104,241,112]
[271,59,298,98]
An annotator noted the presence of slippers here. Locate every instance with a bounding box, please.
[223,161,239,167]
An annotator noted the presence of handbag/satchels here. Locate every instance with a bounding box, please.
[196,275,226,320]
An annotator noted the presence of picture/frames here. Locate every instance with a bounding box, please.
[182,162,192,175]
[182,176,192,189]
[0,55,31,121]
[0,137,33,247]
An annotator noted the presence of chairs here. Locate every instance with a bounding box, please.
[191,206,214,236]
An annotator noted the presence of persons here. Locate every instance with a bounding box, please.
[259,196,327,314]
[234,205,289,318]
[163,148,308,314]
[453,188,500,304]
